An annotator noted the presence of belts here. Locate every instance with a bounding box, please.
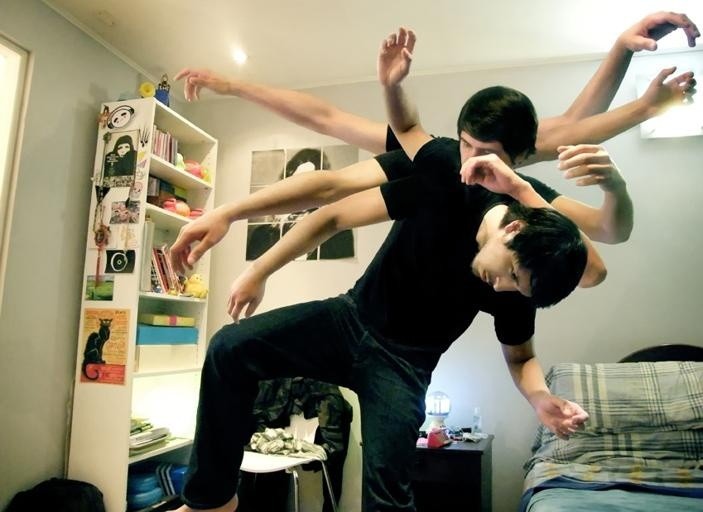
[338,293,427,402]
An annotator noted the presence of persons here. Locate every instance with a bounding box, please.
[166,8,700,511]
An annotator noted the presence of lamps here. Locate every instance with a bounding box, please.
[426,393,451,434]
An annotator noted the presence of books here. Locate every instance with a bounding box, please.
[152,124,179,165]
[128,410,169,457]
[152,248,173,294]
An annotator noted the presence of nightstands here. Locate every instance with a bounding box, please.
[414,432,495,512]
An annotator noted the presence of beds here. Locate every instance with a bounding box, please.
[515,343,703,512]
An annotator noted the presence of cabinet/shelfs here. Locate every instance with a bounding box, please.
[65,96,219,512]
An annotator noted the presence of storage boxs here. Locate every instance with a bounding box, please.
[135,344,199,373]
[136,322,199,344]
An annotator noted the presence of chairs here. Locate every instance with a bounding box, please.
[241,413,338,512]
